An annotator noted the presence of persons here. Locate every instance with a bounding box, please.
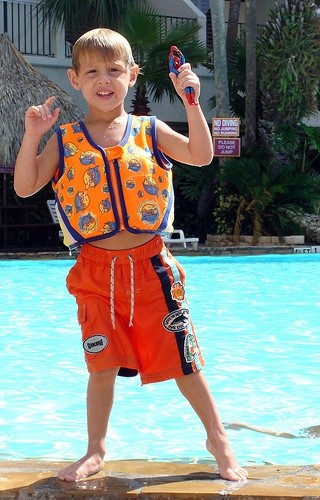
[12,28,250,482]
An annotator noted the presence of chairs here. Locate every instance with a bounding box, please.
[160,230,199,251]
[47,200,74,256]
